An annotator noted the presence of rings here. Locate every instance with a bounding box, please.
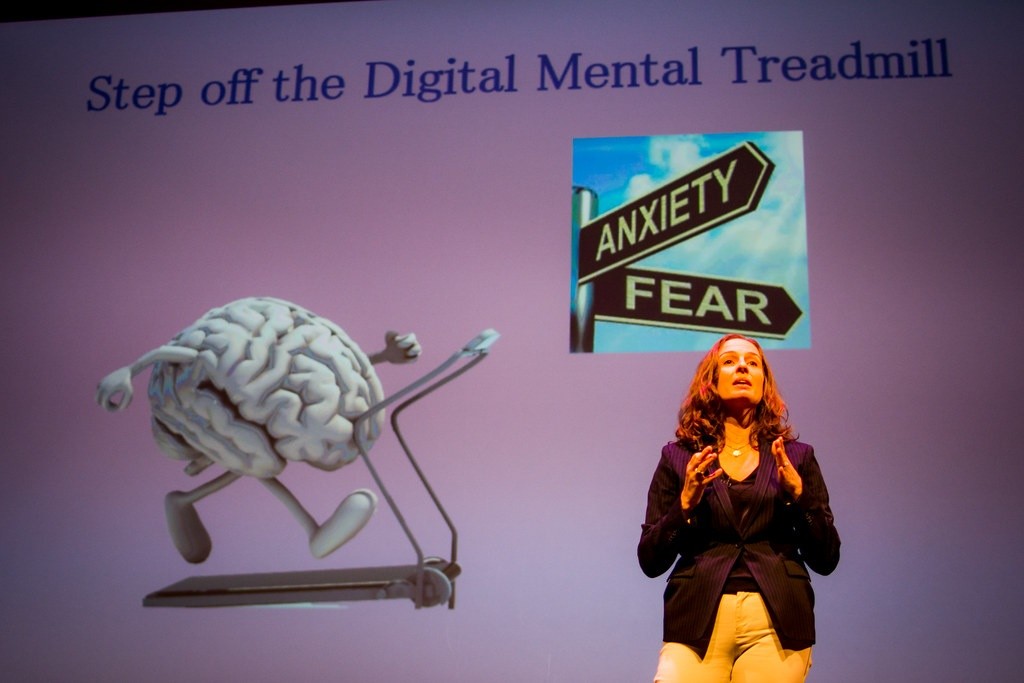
[695,468,704,477]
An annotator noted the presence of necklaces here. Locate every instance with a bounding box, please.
[723,441,750,457]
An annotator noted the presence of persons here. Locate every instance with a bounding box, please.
[637,334,841,683]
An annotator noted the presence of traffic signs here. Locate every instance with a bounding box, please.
[577,140,806,342]
